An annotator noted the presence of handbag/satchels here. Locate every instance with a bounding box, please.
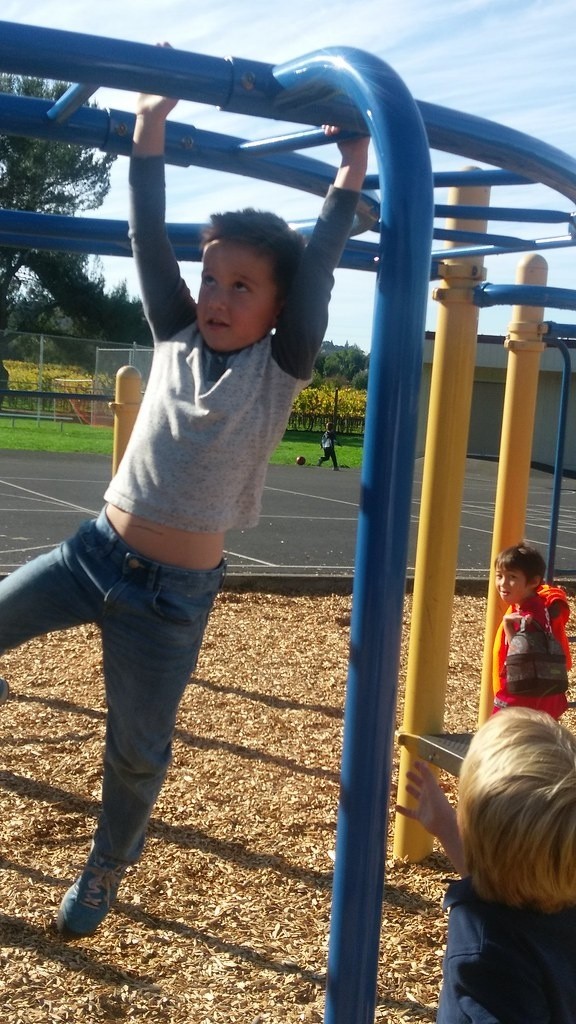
[507,619,568,695]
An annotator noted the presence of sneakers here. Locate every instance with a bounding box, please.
[57,840,132,936]
[0,679,8,707]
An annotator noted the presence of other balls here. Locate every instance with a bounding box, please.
[296,455,308,467]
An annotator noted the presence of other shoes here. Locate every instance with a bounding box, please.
[317,459,322,466]
[333,468,339,471]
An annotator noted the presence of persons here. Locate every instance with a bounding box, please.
[485,543,573,722]
[393,706,576,1024]
[317,421,341,472]
[0,42,374,939]
